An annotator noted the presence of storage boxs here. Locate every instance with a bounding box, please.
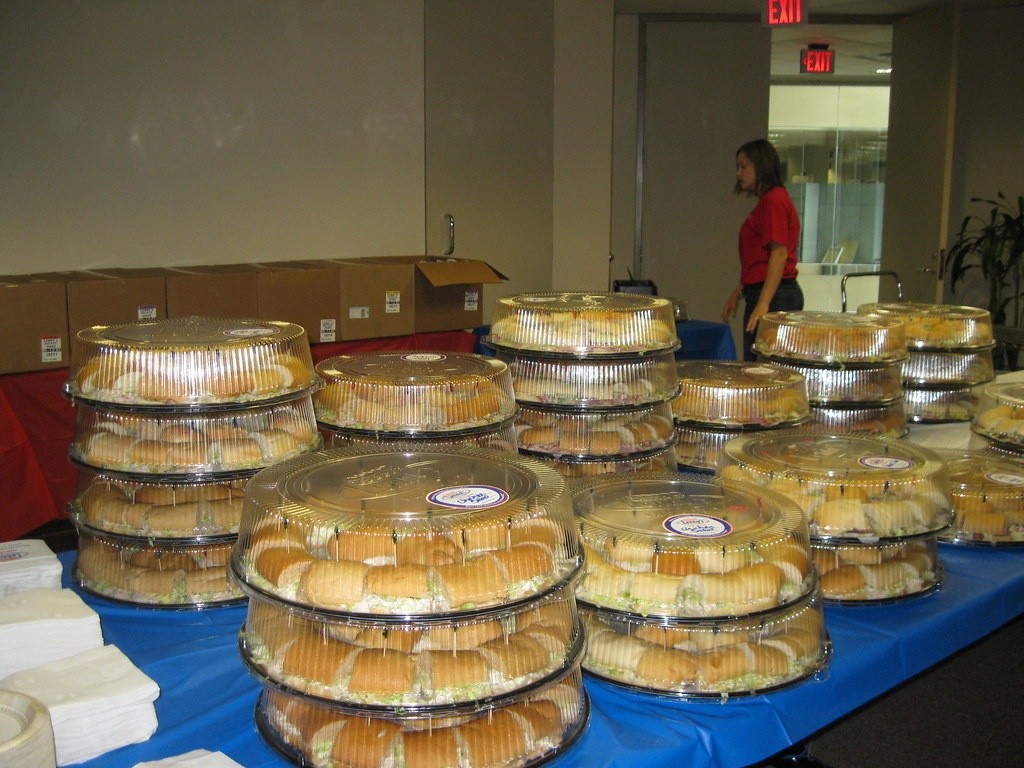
[0,256,510,373]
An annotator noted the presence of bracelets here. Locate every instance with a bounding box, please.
[736,288,742,295]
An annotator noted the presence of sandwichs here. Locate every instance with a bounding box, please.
[70,306,1024,768]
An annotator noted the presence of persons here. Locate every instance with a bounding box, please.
[723,139,804,364]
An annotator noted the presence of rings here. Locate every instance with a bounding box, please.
[756,317,758,319]
